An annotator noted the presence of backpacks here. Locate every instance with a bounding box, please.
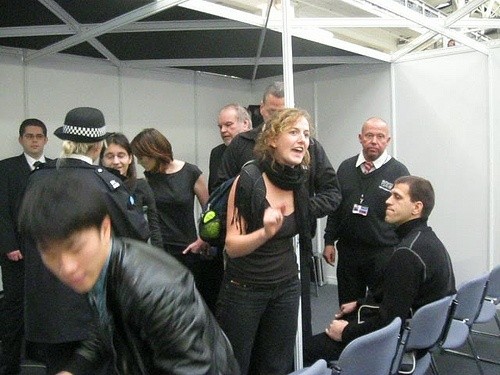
[197,158,263,248]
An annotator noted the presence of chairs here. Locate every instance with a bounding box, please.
[285,265,500,375]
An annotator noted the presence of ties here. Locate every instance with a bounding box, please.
[363,161,374,176]
[32,161,42,171]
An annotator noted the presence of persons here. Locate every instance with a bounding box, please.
[18,170,242,375]
[214,107,316,374]
[0,80,412,375]
[302,175,456,369]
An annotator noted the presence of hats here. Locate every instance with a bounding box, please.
[53,107,115,143]
